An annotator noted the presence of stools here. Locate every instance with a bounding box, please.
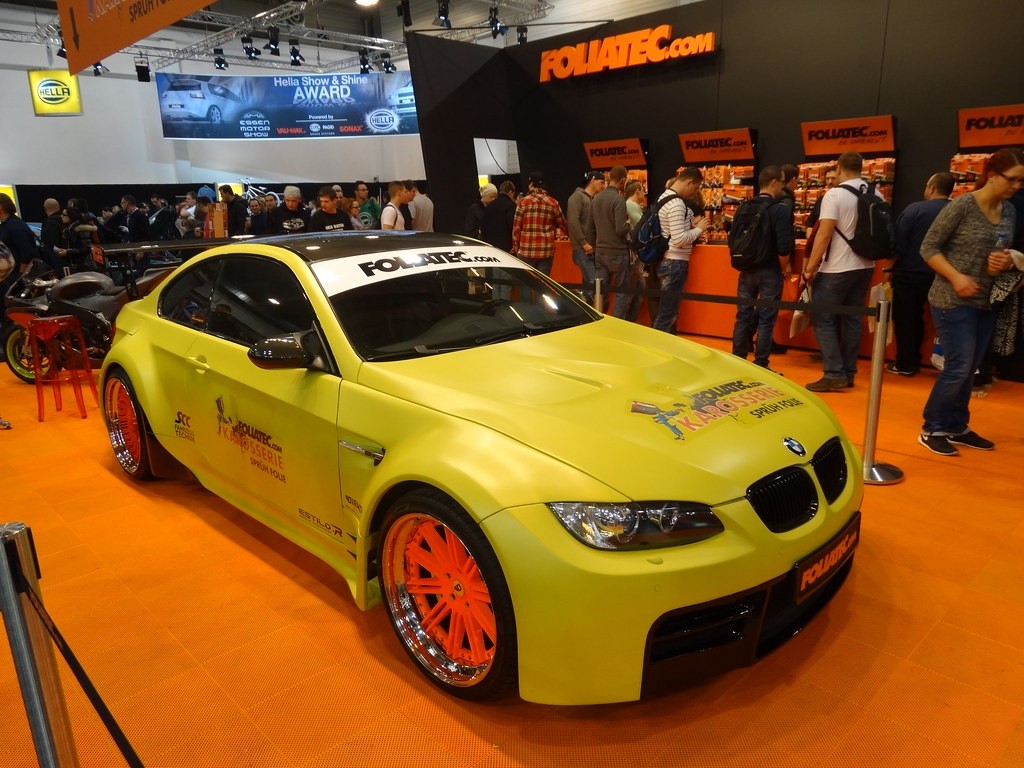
[30,315,100,423]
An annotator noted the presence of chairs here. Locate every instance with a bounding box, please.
[383,285,447,343]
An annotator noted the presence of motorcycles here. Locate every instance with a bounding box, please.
[3,259,172,385]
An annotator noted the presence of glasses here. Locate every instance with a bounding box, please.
[350,206,358,209]
[60,212,68,217]
[778,179,787,185]
[121,201,126,205]
[152,201,157,204]
[996,171,1024,186]
[358,189,368,192]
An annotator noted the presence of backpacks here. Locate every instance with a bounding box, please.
[728,200,783,271]
[634,194,688,267]
[833,182,897,259]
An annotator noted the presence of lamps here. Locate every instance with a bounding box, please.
[488,4,503,40]
[516,25,529,48]
[133,54,152,82]
[92,62,107,76]
[213,44,229,71]
[263,24,281,57]
[380,52,394,75]
[55,30,68,58]
[240,31,261,61]
[436,1,453,27]
[288,38,305,67]
[358,46,371,74]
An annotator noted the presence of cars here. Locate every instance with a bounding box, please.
[98,231,863,704]
[386,80,417,118]
[160,79,244,124]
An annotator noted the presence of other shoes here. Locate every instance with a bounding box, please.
[747,338,787,353]
[767,367,784,376]
[806,375,854,391]
[0,417,10,429]
[808,352,824,362]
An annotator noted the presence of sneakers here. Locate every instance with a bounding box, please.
[945,431,994,450]
[918,434,958,456]
[884,360,921,377]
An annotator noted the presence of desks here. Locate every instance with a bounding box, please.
[96,241,237,301]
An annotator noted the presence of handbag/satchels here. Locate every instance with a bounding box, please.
[38,245,60,271]
[84,243,107,273]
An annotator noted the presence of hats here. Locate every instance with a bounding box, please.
[587,171,605,181]
[480,183,498,196]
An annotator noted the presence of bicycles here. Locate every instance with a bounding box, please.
[241,186,280,207]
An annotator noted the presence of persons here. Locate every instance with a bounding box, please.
[466,152,1024,393]
[0,181,435,362]
[920,148,1024,455]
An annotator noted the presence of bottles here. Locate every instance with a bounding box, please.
[988,232,1007,277]
